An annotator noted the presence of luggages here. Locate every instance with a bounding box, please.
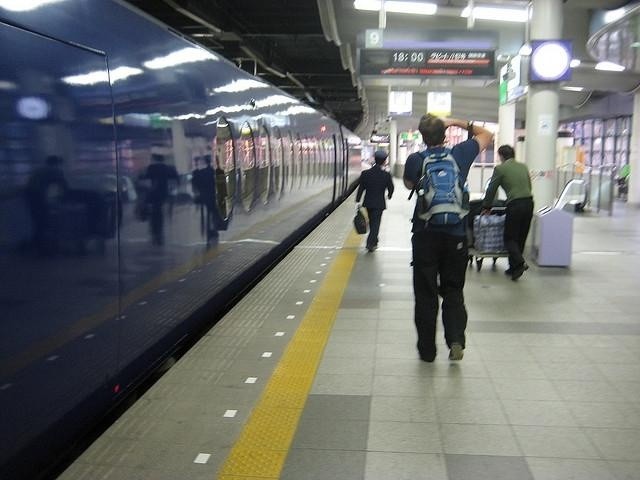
[466,197,507,253]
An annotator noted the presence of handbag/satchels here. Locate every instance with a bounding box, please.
[353,206,369,236]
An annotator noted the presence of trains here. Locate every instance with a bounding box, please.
[2,0,363,479]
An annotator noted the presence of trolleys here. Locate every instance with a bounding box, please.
[466,202,513,273]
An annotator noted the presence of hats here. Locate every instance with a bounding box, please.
[373,151,388,164]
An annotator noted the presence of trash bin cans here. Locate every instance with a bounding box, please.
[532,205,573,267]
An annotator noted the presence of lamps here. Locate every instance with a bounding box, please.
[353,0,438,16]
[460,0,534,23]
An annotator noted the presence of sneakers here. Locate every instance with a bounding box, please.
[503,263,530,282]
[365,244,378,252]
[447,342,465,361]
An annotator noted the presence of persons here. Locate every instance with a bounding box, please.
[354,151,394,252]
[137,153,229,248]
[25,155,70,256]
[481,145,534,280]
[403,114,494,363]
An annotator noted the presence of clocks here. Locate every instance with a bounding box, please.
[528,39,572,83]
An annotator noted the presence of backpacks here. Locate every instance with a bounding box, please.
[415,149,472,229]
